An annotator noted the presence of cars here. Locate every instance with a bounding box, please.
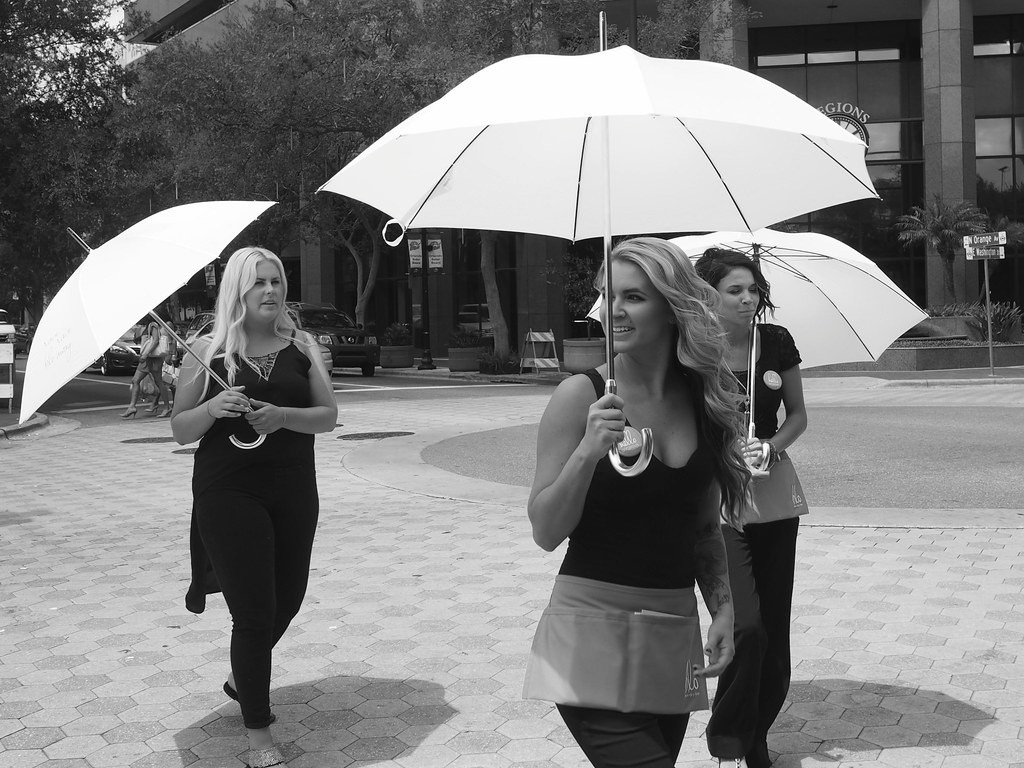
[287,307,381,377]
[0,308,219,376]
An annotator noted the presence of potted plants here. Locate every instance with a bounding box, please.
[447,324,493,371]
[379,321,415,368]
[538,252,606,373]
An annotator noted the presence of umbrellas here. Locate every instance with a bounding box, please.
[585,229,929,475]
[19,201,278,450]
[315,46,883,476]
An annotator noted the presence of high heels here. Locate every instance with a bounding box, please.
[145,404,159,414]
[120,406,137,419]
[157,406,172,418]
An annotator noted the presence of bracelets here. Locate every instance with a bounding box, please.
[764,440,783,470]
[280,406,288,426]
[207,400,214,418]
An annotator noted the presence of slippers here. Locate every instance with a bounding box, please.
[248,746,289,768]
[224,681,275,723]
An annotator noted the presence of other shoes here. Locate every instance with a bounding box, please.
[719,756,749,768]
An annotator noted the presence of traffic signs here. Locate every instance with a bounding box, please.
[961,231,1007,260]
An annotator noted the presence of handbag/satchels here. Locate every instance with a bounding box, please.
[140,322,169,358]
[129,372,160,395]
[161,360,180,385]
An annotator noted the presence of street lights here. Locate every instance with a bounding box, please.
[998,166,1009,192]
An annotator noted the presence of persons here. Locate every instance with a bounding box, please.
[170,246,338,768]
[119,306,176,419]
[692,247,808,768]
[523,236,753,768]
